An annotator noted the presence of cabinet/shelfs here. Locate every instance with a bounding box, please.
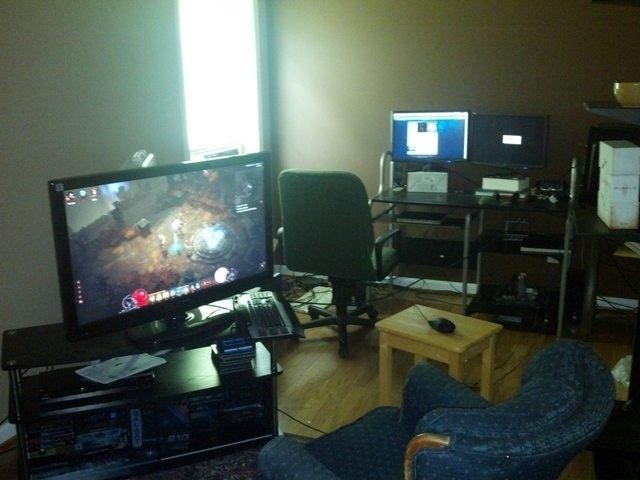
[0,295,279,480]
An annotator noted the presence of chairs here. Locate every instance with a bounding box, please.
[276,169,402,357]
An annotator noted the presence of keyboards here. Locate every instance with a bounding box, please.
[235,290,307,346]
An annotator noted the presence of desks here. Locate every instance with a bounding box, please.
[367,151,579,339]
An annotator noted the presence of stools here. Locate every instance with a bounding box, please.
[375,304,502,407]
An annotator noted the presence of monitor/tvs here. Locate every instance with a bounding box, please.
[47,150,274,354]
[390,108,470,172]
[470,112,551,177]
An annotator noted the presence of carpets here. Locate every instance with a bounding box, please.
[114,450,263,480]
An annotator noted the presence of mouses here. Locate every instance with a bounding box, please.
[427,316,458,335]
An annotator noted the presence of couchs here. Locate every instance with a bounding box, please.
[256,339,615,479]
